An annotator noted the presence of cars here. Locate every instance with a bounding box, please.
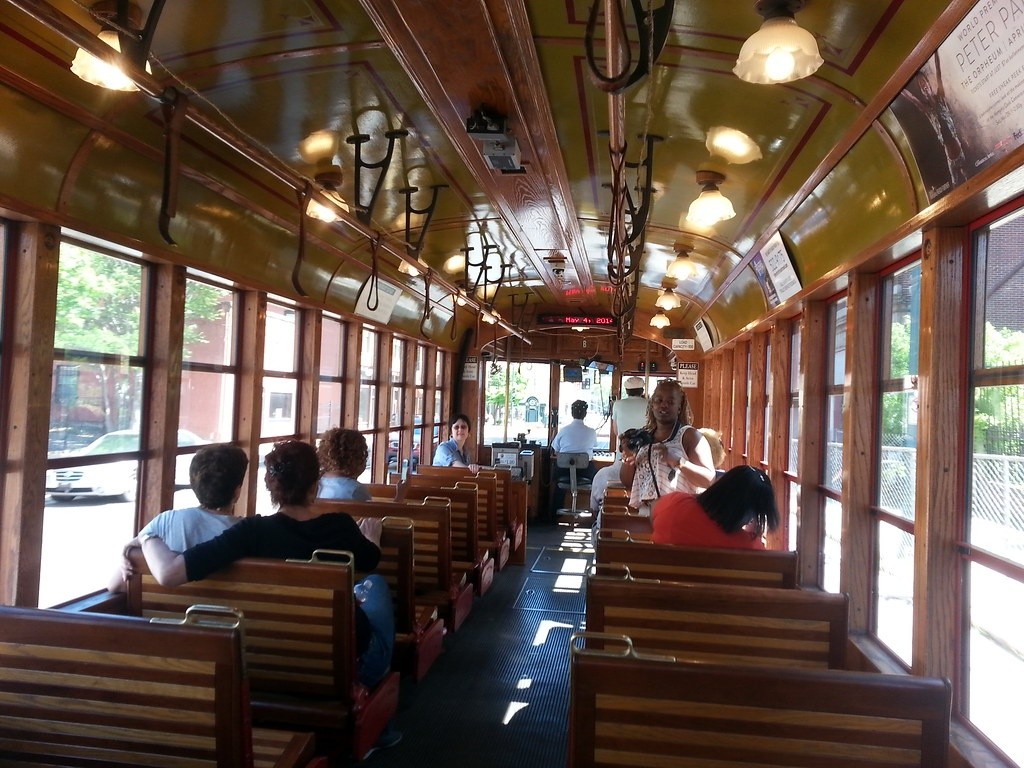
[45,429,215,502]
[387,418,440,470]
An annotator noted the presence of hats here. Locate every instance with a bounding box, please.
[625,377,644,389]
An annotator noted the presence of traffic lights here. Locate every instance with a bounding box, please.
[582,370,600,389]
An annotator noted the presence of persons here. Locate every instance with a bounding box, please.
[652,465,780,550]
[591,429,639,549]
[122,441,403,759]
[315,428,374,501]
[432,413,479,474]
[107,443,248,596]
[551,399,598,528]
[620,378,726,516]
[612,377,649,436]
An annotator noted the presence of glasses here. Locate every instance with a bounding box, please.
[453,425,467,430]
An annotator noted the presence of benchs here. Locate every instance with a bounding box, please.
[0,465,528,768]
[569,479,953,768]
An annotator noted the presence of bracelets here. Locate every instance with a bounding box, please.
[673,458,687,472]
[622,459,635,466]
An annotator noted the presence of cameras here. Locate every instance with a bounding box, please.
[623,430,652,451]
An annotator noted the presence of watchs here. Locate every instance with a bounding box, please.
[140,532,162,546]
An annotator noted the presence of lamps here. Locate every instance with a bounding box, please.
[305,173,349,223]
[654,282,681,311]
[453,279,467,306]
[649,307,671,329]
[674,212,718,249]
[394,211,424,243]
[732,0,825,85]
[698,125,762,175]
[477,284,497,304]
[296,129,341,175]
[685,171,738,224]
[481,303,501,325]
[398,242,429,276]
[70,0,152,93]
[442,255,465,280]
[666,243,699,280]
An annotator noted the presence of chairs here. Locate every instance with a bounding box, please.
[556,452,592,532]
